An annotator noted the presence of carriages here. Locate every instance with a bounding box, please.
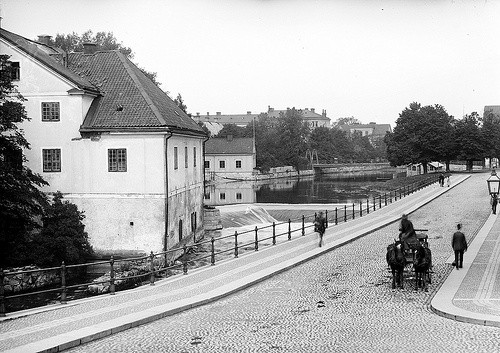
[386,228,432,293]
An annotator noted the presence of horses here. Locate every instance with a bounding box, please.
[386,239,407,292]
[406,243,432,293]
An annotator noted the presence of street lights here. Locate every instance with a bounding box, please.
[487,169,500,213]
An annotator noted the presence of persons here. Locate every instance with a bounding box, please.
[443,173,451,188]
[487,189,499,215]
[438,170,446,185]
[392,214,425,260]
[450,224,468,270]
[313,210,328,248]
